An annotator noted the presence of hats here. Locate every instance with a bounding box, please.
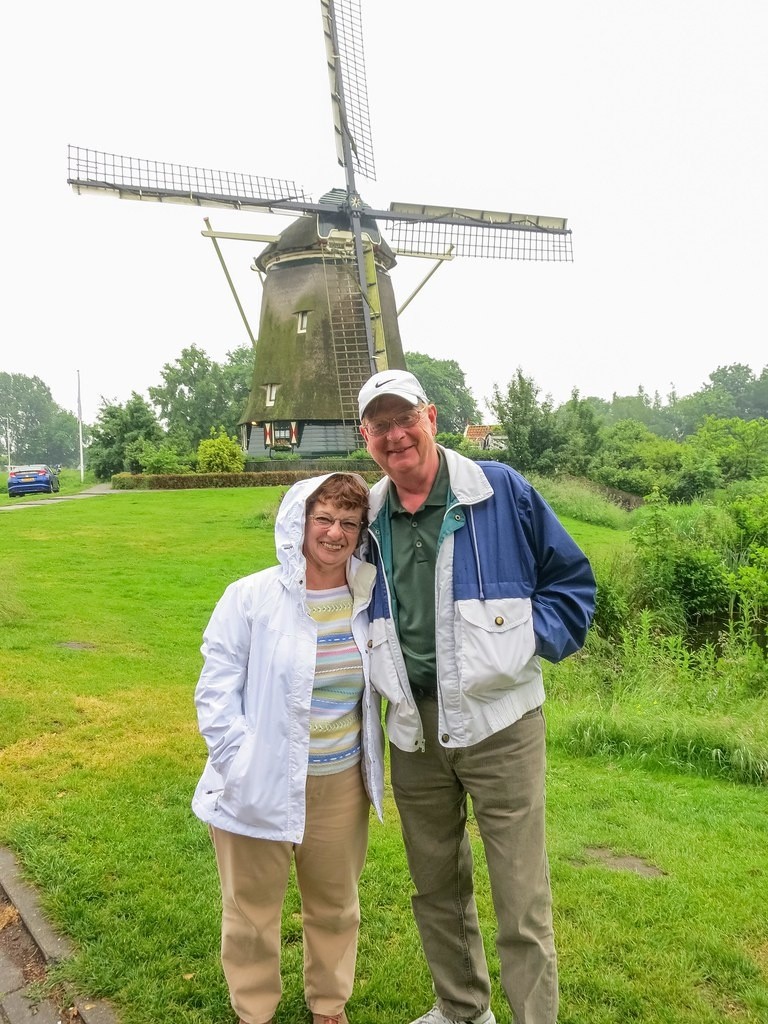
[357,369,430,424]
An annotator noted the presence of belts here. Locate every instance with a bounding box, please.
[411,683,437,697]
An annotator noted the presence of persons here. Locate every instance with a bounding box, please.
[357,370,597,1024]
[192,472,386,1024]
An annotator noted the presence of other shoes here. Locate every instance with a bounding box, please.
[238,1018,272,1024]
[313,1009,349,1024]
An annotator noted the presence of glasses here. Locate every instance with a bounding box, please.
[306,511,366,533]
[362,406,426,436]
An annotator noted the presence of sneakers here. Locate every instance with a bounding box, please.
[408,1006,496,1024]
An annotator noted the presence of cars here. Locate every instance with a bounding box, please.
[8,464,60,497]
